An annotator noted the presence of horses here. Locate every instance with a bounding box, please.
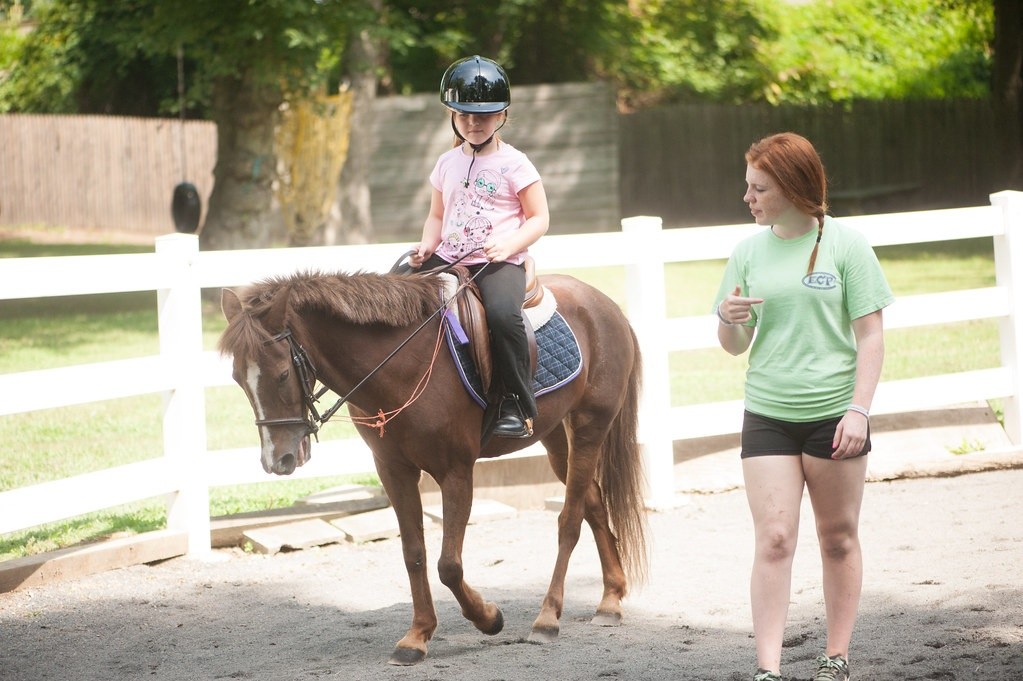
[215,273,647,666]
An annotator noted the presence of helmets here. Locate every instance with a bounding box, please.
[440,55,511,113]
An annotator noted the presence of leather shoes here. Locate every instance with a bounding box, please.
[493,410,527,436]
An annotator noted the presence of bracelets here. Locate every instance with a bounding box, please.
[846,404,871,419]
[715,300,734,327]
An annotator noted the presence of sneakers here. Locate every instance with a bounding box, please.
[813,652,850,681]
[753,667,782,681]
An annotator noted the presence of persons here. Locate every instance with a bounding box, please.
[711,132,893,681]
[387,58,551,437]
